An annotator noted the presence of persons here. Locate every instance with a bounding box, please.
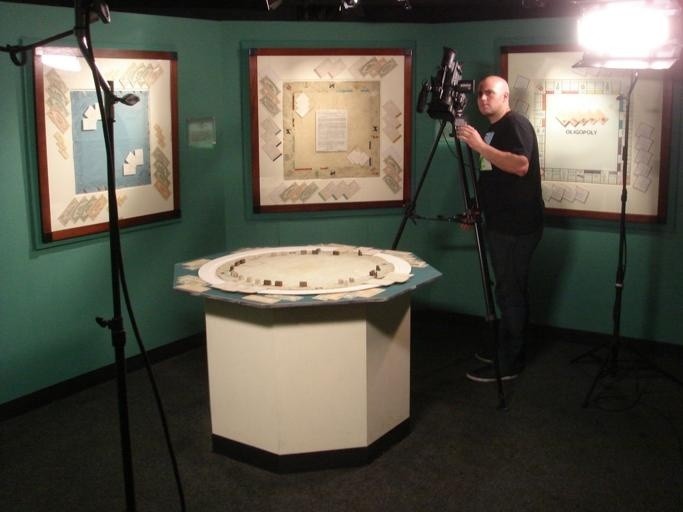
[456,75,545,382]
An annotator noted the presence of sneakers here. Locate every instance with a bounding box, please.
[475,351,496,364]
[466,365,519,382]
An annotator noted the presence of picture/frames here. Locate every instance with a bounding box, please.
[247,44,414,214]
[500,43,674,224]
[29,42,183,243]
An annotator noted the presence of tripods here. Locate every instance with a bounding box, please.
[390,116,508,408]
